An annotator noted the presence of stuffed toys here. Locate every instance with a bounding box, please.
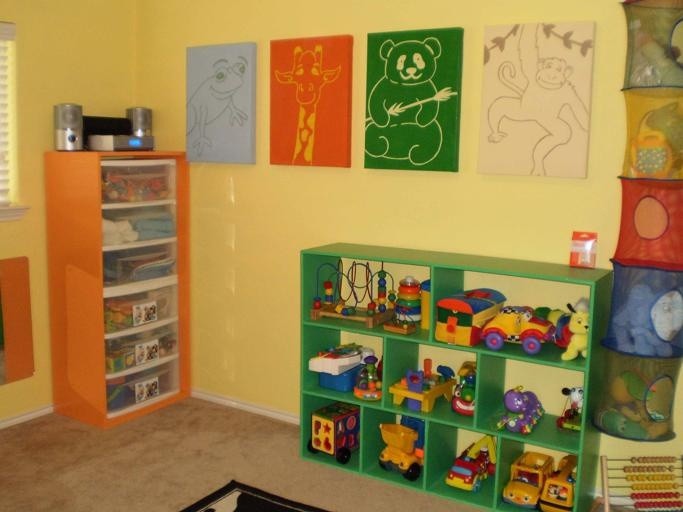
[560,298,590,361]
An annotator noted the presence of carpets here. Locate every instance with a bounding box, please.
[1,398,491,512]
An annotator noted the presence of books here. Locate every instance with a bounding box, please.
[593,361,677,439]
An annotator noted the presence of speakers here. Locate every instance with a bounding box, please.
[53,101,83,152]
[127,107,153,136]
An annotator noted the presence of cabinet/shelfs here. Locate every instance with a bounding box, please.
[297,243,613,512]
[41,148,192,431]
